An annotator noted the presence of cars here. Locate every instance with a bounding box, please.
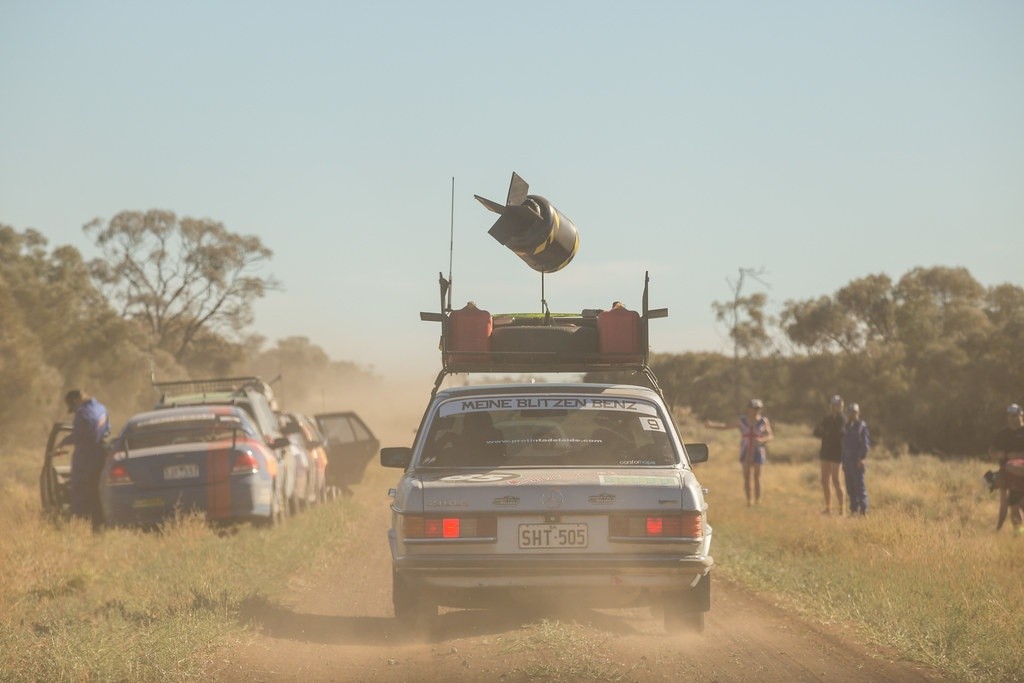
[380,352,713,628]
[153,375,381,516]
[40,401,290,533]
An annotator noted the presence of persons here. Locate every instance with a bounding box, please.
[705,399,774,509]
[460,411,504,457]
[813,396,846,517]
[840,402,869,518]
[52,388,111,535]
[993,403,1024,534]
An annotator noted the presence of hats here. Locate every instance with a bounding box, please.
[848,402,859,411]
[750,399,763,408]
[829,394,844,407]
[66,390,80,413]
[1007,403,1019,413]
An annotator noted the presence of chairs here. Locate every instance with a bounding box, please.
[447,413,509,466]
[574,426,640,465]
